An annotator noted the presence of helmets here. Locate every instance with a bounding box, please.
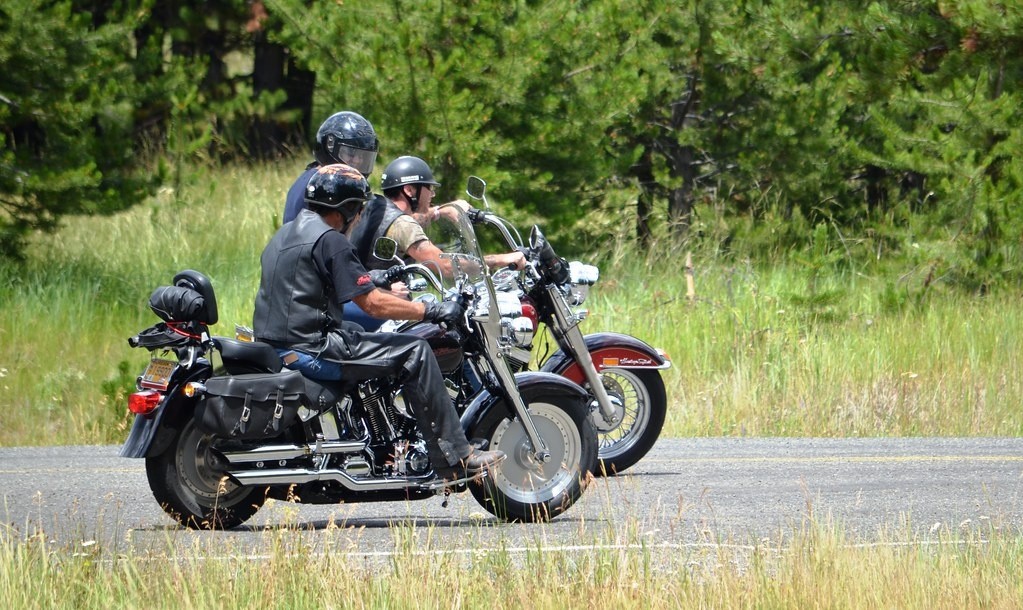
[380,156,443,190]
[316,111,379,178]
[303,163,373,222]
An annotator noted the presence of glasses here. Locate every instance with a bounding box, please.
[422,184,436,193]
[342,147,356,156]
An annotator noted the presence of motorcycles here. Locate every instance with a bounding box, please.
[118,203,599,530]
[234,176,671,477]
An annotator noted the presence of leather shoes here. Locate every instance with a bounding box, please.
[437,449,507,479]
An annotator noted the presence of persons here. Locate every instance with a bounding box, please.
[252,163,507,479]
[283,112,408,333]
[349,157,528,275]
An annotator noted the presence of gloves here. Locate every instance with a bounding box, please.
[423,299,465,328]
[368,270,393,293]
